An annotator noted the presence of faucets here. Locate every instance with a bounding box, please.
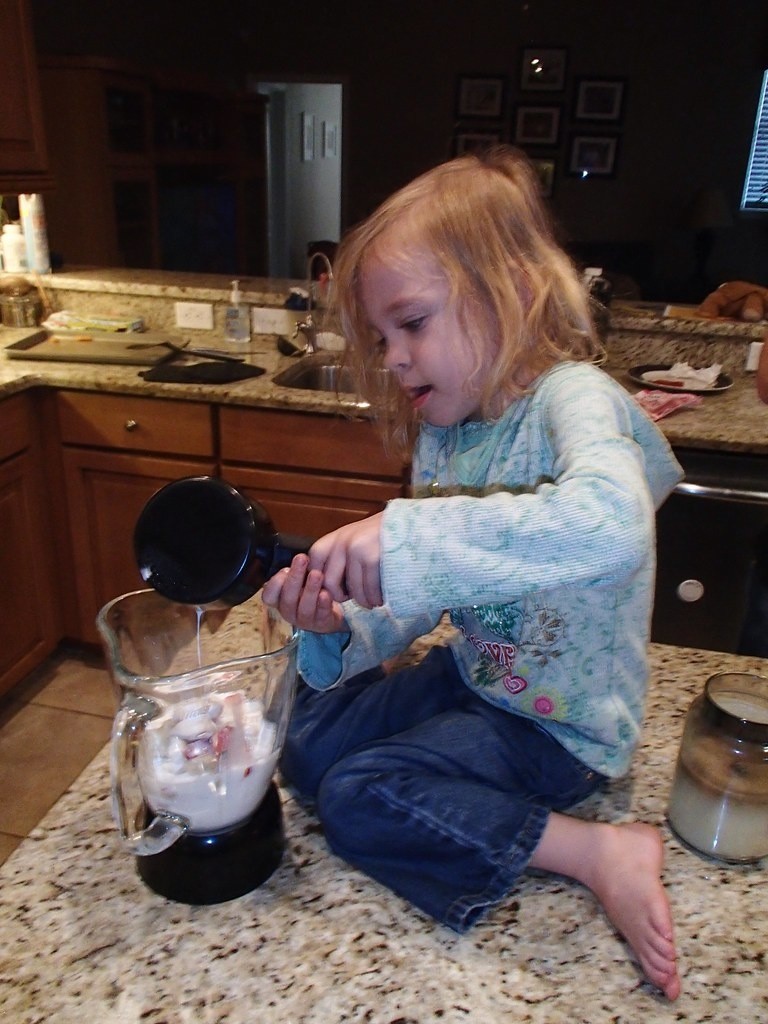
[305,252,334,310]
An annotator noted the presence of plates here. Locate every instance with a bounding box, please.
[626,363,735,394]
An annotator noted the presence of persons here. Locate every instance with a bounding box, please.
[260,142,682,1001]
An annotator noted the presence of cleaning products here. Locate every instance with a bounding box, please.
[224,280,250,342]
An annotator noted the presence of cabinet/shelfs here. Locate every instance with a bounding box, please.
[0,385,768,697]
[35,54,269,275]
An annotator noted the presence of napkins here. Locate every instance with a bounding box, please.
[643,361,723,389]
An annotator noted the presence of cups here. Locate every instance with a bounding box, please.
[135,475,349,611]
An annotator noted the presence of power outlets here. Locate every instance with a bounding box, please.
[176,302,213,329]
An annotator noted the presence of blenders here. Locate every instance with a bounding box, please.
[96,586,301,908]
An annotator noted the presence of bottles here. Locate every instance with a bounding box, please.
[1,223,27,273]
[665,671,768,871]
[582,268,612,348]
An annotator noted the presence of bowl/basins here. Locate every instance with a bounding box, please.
[1,286,42,328]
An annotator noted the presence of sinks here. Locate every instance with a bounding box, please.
[271,351,403,395]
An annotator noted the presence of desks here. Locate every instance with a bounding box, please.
[0,575,767,1024]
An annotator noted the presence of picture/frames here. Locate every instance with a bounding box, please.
[562,130,622,179]
[511,100,564,149]
[571,75,629,127]
[323,121,336,159]
[452,73,507,121]
[515,44,572,93]
[301,110,316,163]
[452,126,504,159]
[525,154,558,201]
